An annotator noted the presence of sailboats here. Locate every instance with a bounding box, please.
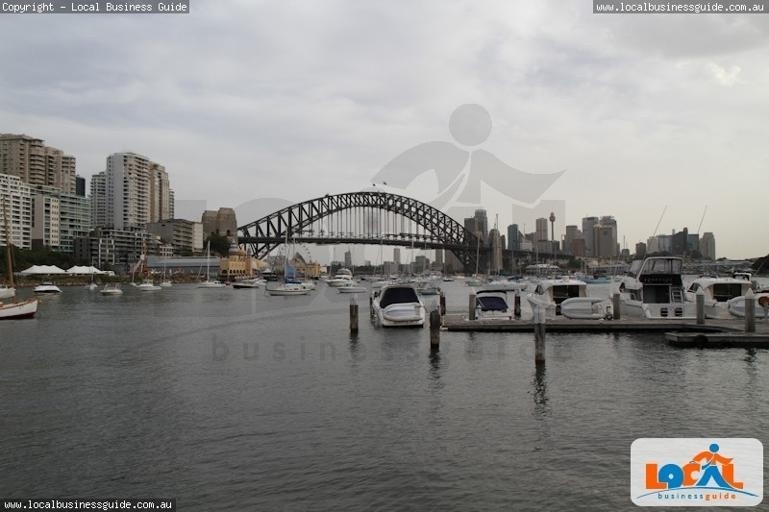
[325,246,367,293]
[160,270,172,288]
[372,236,454,293]
[197,240,226,287]
[136,272,163,290]
[265,227,317,295]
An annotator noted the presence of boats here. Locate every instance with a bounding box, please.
[100,283,122,294]
[686,272,769,320]
[471,284,514,322]
[613,256,698,321]
[527,279,607,319]
[373,284,427,329]
[33,283,63,293]
[232,278,259,288]
[0,298,38,318]
